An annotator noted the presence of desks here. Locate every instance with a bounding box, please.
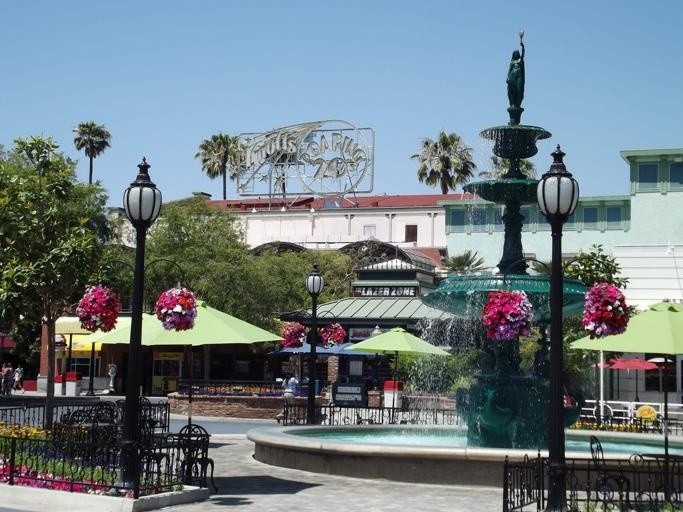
[642,454,683,512]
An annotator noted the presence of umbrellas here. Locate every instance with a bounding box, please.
[79,313,152,343]
[569,297,683,502]
[93,296,289,435]
[326,343,386,357]
[344,325,454,422]
[590,357,629,400]
[610,355,660,404]
[266,341,334,354]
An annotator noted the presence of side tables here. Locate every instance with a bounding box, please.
[610,416,630,426]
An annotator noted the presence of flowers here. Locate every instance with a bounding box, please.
[580,283,633,340]
[278,322,299,346]
[75,290,116,330]
[154,279,197,332]
[483,288,534,345]
[324,320,343,346]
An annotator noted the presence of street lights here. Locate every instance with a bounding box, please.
[536,144,579,512]
[305,264,323,424]
[115,158,162,489]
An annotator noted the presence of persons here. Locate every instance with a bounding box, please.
[0,361,27,397]
[275,369,301,423]
[363,364,376,401]
[506,41,525,107]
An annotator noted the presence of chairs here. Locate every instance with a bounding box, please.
[635,405,663,434]
[589,435,631,512]
[59,396,219,493]
[593,403,613,428]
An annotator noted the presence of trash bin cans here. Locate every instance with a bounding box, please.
[302,386,309,396]
[384,382,404,412]
[55,373,83,396]
[315,380,322,395]
